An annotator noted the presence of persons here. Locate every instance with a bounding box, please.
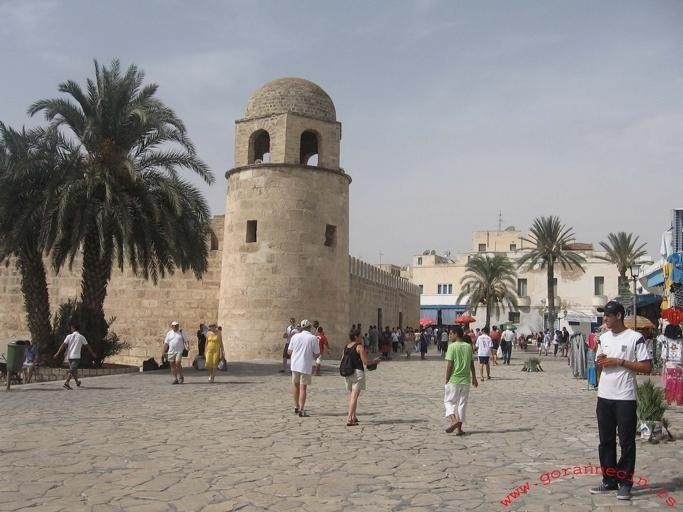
[433,326,437,344]
[426,325,432,341]
[349,324,356,340]
[314,326,330,376]
[420,329,427,359]
[537,331,545,356]
[196,322,204,357]
[368,324,372,346]
[403,326,411,359]
[396,325,401,337]
[372,325,378,352]
[476,328,481,338]
[204,323,223,383]
[312,320,319,335]
[469,329,474,338]
[21,340,38,385]
[414,329,421,352]
[288,321,320,418]
[499,325,514,365]
[553,330,559,357]
[543,330,549,355]
[558,331,563,348]
[381,326,391,359]
[391,328,399,354]
[362,332,369,350]
[441,329,447,357]
[546,328,551,339]
[490,325,500,365]
[561,327,569,357]
[355,322,361,337]
[280,318,295,370]
[518,333,527,351]
[342,329,380,425]
[444,326,478,436]
[291,323,300,336]
[471,328,493,380]
[52,325,96,389]
[497,325,504,337]
[463,327,468,341]
[589,302,652,498]
[161,321,189,384]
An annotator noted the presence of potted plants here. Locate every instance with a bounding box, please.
[637,379,666,441]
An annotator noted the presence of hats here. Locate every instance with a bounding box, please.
[171,321,179,326]
[300,319,312,328]
[597,299,625,315]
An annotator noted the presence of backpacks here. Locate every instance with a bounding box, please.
[339,342,361,375]
[179,329,187,358]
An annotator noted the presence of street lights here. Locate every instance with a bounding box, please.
[628,261,640,332]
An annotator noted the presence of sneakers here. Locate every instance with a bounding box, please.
[63,382,73,390]
[178,377,184,384]
[171,379,178,384]
[589,482,619,494]
[615,484,631,499]
[298,410,310,418]
[295,407,299,413]
[77,379,82,387]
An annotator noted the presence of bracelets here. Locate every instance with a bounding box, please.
[621,359,625,367]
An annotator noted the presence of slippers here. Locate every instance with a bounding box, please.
[445,421,460,434]
[355,418,359,422]
[456,430,465,436]
[347,422,359,426]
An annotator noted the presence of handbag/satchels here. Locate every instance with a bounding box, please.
[180,329,188,357]
[217,353,227,372]
